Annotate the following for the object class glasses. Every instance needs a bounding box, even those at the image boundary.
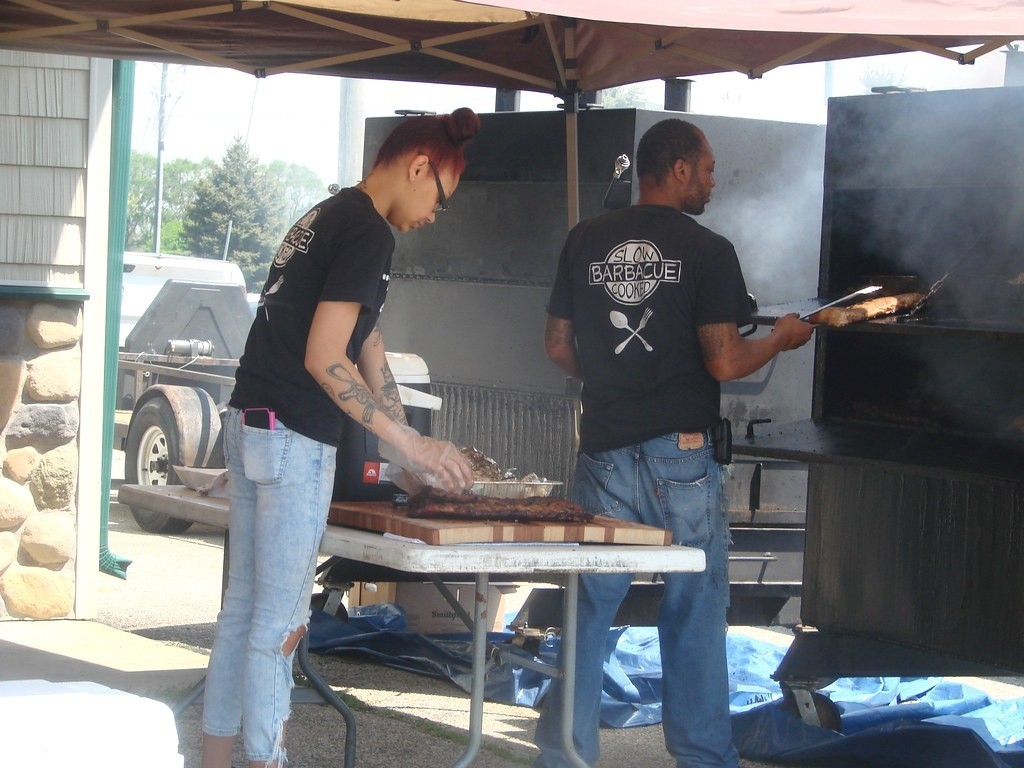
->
[418,151,450,213]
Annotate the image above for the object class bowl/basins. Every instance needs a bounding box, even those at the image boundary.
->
[171,464,227,493]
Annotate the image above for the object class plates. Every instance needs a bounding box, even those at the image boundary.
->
[471,479,563,498]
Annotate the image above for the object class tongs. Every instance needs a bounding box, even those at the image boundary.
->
[770,284,882,333]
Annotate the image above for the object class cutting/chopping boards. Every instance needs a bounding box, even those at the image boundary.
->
[324,499,673,547]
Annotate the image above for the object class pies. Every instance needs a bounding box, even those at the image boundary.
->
[808,293,924,326]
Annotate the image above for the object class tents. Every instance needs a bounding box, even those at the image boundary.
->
[0,0,1024,495]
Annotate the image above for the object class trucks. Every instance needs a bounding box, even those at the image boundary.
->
[114,253,256,533]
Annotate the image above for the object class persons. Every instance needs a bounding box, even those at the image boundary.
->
[535,120,815,768]
[199,107,480,768]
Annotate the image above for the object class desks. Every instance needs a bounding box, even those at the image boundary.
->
[116,485,708,768]
